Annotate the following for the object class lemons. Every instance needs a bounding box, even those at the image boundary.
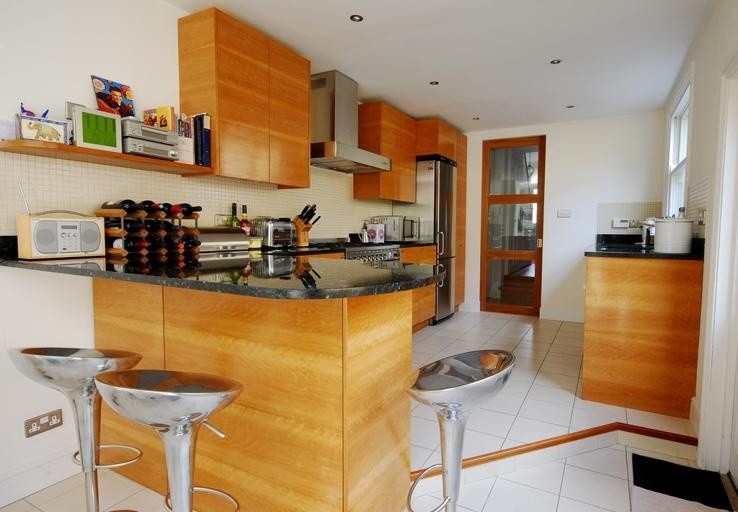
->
[357,229,372,244]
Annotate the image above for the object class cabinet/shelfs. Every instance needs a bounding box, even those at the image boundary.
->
[100,197,203,273]
[676,205,687,220]
[228,261,251,289]
[230,202,252,234]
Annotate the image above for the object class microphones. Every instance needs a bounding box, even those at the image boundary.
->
[19,346,242,510]
[405,350,517,512]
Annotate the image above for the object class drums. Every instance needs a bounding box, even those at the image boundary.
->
[295,269,322,291]
[298,202,322,228]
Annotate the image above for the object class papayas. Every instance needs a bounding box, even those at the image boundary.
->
[253,214,298,249]
[252,254,298,280]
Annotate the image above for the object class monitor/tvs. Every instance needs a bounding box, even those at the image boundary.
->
[202,115,211,168]
[194,116,203,167]
[142,106,195,138]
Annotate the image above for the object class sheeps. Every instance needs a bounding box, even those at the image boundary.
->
[634,223,651,248]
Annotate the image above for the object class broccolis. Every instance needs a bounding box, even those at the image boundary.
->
[392,153,457,327]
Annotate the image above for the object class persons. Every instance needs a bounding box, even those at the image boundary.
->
[96,86,134,117]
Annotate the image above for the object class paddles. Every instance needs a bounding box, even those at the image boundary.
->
[631,452,737,512]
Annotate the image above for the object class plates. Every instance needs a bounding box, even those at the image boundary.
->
[580,256,704,419]
[352,101,418,204]
[176,7,312,188]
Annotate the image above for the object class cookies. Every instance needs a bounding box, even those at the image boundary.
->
[370,214,420,241]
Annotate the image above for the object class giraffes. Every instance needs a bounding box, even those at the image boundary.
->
[316,241,401,260]
